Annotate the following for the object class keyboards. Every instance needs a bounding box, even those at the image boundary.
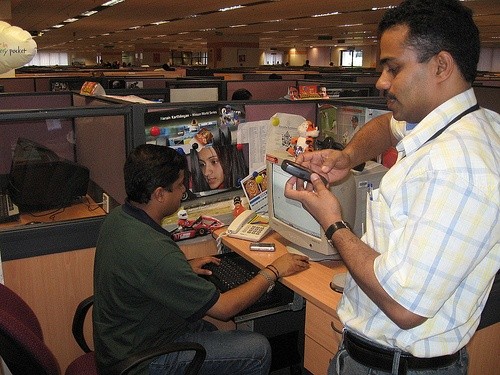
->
[0,194,20,224]
[198,253,281,313]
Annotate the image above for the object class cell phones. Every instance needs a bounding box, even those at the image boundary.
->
[281,159,327,187]
[250,242,275,252]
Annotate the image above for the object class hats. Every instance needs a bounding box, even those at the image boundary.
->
[233,196,242,204]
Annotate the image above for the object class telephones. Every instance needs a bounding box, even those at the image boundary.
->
[227,209,271,242]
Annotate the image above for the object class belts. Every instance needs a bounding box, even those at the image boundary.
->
[343,330,461,375]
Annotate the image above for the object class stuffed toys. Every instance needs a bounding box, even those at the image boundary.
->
[286,120,319,157]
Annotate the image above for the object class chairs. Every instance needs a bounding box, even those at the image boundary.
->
[0,283,206,375]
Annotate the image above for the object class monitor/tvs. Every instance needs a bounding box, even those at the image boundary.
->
[7,137,91,212]
[265,148,388,260]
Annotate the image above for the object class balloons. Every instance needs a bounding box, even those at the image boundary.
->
[0,20,37,75]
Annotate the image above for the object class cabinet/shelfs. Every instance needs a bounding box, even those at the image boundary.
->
[212,218,500,375]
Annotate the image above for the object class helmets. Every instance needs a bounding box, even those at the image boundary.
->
[178,210,188,220]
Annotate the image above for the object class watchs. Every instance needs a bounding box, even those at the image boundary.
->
[258,269,275,293]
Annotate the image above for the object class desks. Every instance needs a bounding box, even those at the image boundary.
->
[0,195,107,375]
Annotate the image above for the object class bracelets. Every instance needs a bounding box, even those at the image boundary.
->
[325,220,352,249]
[265,265,280,280]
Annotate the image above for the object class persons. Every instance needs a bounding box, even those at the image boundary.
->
[99,59,132,69]
[274,61,289,67]
[176,210,196,232]
[302,60,310,71]
[231,88,253,101]
[285,0,500,375]
[329,62,333,67]
[162,63,176,71]
[317,86,329,99]
[190,144,249,193]
[343,115,361,144]
[91,144,311,375]
[288,85,302,99]
[233,196,245,219]
